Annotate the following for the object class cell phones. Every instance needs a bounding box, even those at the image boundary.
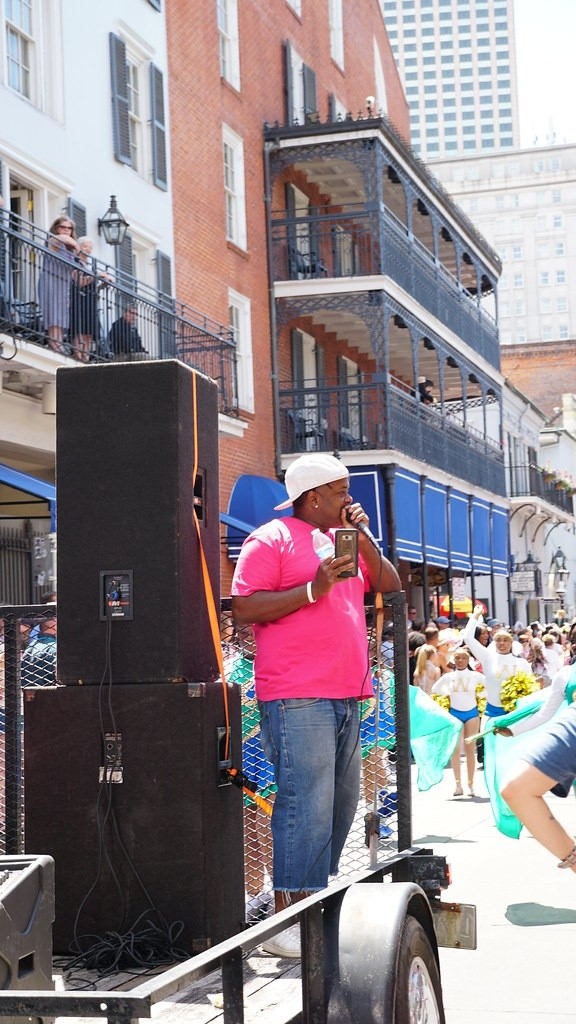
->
[335,529,358,578]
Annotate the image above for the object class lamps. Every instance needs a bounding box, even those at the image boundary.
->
[97,195,129,245]
[41,383,56,415]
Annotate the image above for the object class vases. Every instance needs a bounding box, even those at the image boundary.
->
[544,474,575,497]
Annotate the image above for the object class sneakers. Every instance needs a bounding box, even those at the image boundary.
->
[262,922,301,957]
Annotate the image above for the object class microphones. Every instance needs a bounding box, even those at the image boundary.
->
[345,508,381,553]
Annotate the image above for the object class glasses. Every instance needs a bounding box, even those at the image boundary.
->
[410,612,416,615]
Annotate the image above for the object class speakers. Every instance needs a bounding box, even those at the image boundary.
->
[57,360,222,682]
[0,854,56,1024]
[23,681,246,966]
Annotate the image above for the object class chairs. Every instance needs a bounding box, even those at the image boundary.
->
[291,247,328,279]
[286,409,376,452]
[0,281,109,362]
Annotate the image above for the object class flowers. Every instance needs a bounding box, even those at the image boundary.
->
[542,459,576,493]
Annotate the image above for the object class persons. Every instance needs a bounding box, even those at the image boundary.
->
[227,451,401,957]
[109,303,156,362]
[409,378,435,459]
[0,601,576,844]
[500,700,576,875]
[39,217,112,363]
[225,612,280,925]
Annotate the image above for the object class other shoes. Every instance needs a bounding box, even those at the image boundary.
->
[467,781,475,796]
[454,779,463,796]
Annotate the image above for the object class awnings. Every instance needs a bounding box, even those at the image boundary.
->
[0,463,57,533]
[227,472,294,564]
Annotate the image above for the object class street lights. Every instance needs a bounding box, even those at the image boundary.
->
[555,546,570,626]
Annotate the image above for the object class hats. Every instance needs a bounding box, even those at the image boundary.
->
[437,616,451,623]
[488,619,503,627]
[274,454,349,510]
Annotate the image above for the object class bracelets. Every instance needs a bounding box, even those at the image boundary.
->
[306,580,316,603]
[558,848,576,869]
